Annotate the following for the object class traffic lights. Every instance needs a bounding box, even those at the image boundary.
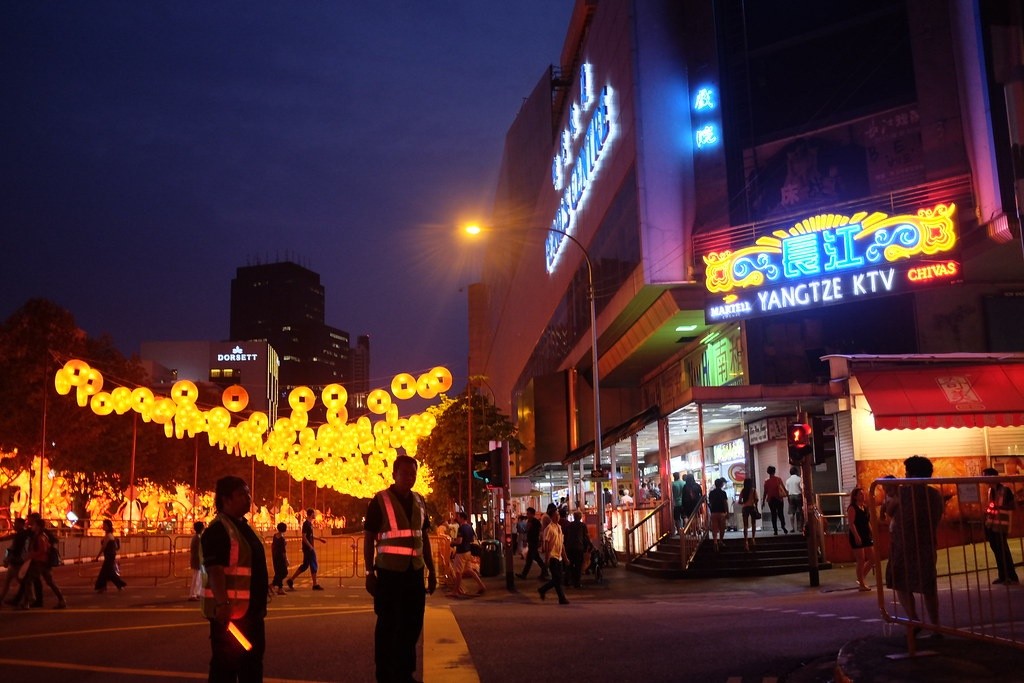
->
[810,415,836,465]
[785,421,814,467]
[471,447,503,489]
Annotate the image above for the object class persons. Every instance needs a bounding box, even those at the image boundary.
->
[448,512,487,595]
[95,519,128,593]
[603,487,612,505]
[0,512,67,609]
[477,515,505,552]
[268,522,289,595]
[671,472,702,536]
[555,497,568,508]
[785,468,804,532]
[190,521,204,601]
[617,484,633,504]
[640,481,661,501]
[882,456,944,639]
[287,509,327,590]
[512,503,593,604]
[708,477,729,552]
[761,466,788,534]
[847,488,875,591]
[738,478,759,546]
[201,476,268,683]
[364,456,437,683]
[983,468,1019,585]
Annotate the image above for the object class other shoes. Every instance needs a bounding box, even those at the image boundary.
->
[538,586,546,601]
[855,578,871,592]
[188,595,200,602]
[559,600,570,606]
[914,626,946,639]
[268,579,295,596]
[97,586,108,594]
[744,538,757,552]
[54,600,65,610]
[312,584,325,590]
[774,532,778,535]
[713,540,726,554]
[790,529,795,533]
[781,526,788,534]
[515,572,526,578]
[118,583,128,593]
[475,587,488,595]
[537,572,550,580]
[574,584,581,588]
[445,592,461,598]
[0,597,45,609]
[992,577,1020,585]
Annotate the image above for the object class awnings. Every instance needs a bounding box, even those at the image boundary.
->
[854,363,1024,431]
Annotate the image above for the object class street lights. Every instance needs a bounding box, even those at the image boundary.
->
[461,217,607,566]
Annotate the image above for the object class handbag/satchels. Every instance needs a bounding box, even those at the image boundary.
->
[115,538,120,550]
[470,543,482,556]
[752,500,762,519]
[777,482,787,498]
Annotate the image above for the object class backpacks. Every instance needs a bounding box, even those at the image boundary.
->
[43,533,63,568]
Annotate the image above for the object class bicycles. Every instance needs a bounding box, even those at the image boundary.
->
[599,525,619,569]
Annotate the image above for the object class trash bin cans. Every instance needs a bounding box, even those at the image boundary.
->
[480,541,501,577]
